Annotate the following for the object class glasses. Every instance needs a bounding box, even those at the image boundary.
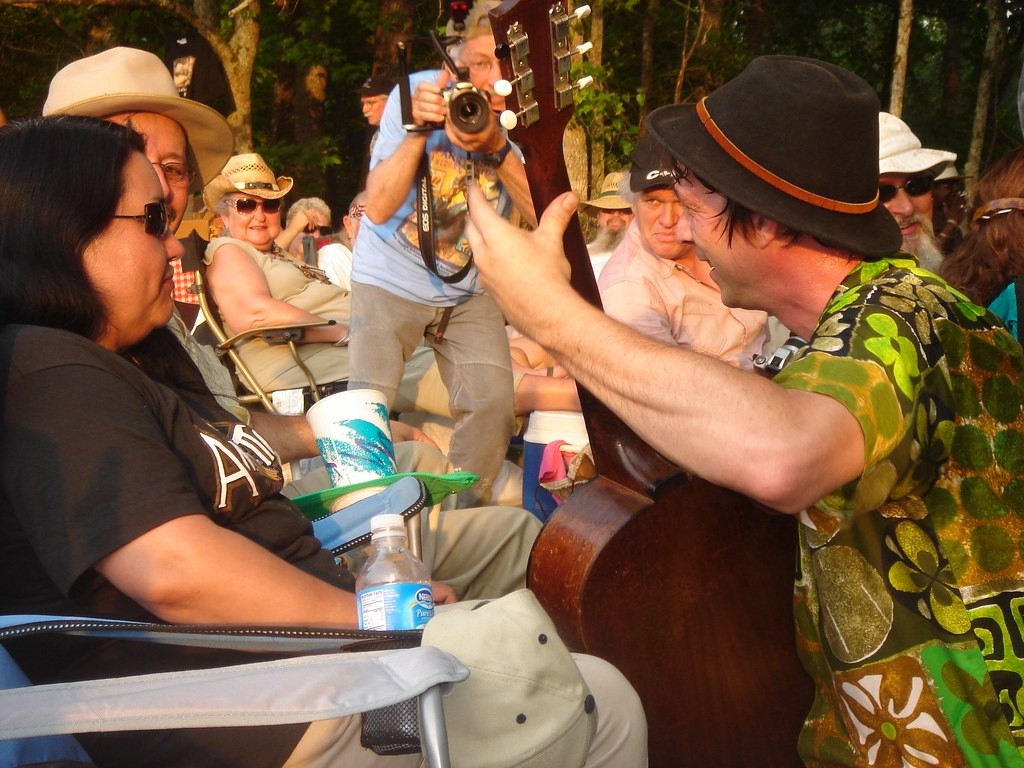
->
[150,161,196,190]
[878,174,938,202]
[113,201,169,238]
[223,198,281,215]
[601,208,633,217]
[302,223,333,237]
[935,179,957,186]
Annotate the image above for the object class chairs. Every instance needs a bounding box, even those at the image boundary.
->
[196,268,338,416]
[0,456,482,767]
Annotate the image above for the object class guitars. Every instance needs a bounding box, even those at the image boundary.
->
[483,0,815,768]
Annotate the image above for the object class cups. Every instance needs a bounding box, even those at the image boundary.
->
[306,389,398,489]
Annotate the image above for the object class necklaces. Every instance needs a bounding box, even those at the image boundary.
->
[262,241,331,285]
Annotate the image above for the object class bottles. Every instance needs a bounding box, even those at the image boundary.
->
[357,514,435,753]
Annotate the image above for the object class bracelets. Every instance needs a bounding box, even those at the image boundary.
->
[334,329,350,346]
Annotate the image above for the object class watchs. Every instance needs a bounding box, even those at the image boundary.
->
[485,140,512,167]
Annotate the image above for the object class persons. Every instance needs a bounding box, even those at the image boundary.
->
[200,153,634,502]
[466,58,1024,768]
[0,116,648,768]
[879,112,1023,348]
[347,0,538,509]
[44,47,543,601]
[599,137,806,370]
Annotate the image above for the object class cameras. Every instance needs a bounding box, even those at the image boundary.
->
[427,66,492,134]
[752,330,810,381]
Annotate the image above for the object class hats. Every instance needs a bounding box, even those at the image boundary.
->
[203,153,294,214]
[578,172,632,218]
[422,587,597,768]
[924,160,974,181]
[353,76,393,96]
[444,0,505,56]
[43,47,235,195]
[877,110,958,179]
[630,139,675,195]
[646,54,903,260]
